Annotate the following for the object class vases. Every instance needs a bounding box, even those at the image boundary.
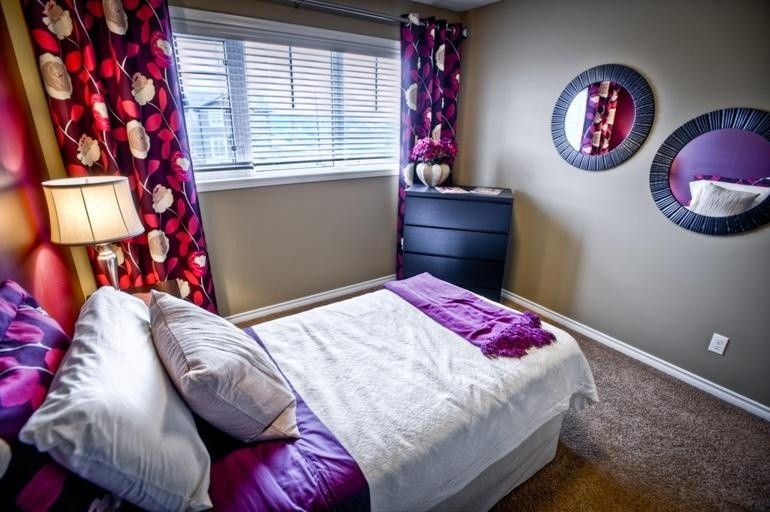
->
[415,163,451,192]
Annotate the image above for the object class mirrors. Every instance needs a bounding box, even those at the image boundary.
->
[549,62,770,236]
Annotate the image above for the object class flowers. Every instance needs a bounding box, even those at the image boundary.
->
[408,136,460,163]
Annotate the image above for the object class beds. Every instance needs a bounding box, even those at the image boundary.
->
[120,272,585,510]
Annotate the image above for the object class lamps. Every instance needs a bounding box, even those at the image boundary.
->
[41,176,147,291]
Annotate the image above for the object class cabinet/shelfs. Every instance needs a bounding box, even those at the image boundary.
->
[401,185,514,302]
